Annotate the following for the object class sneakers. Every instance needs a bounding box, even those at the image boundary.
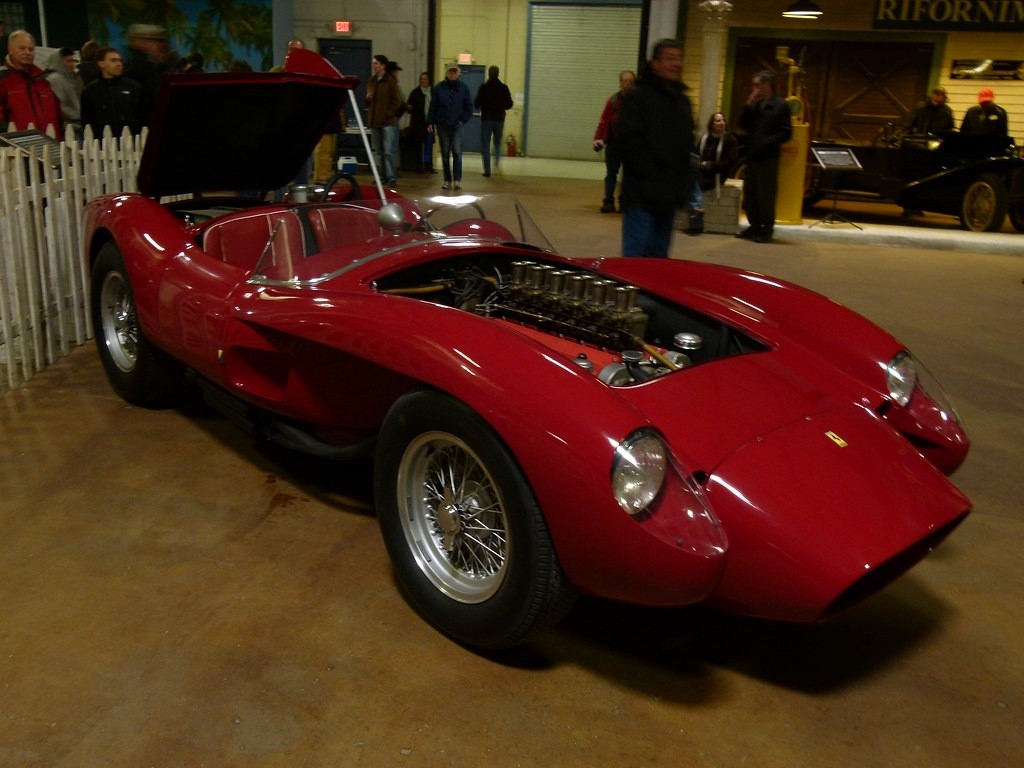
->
[442,181,451,189]
[455,180,461,189]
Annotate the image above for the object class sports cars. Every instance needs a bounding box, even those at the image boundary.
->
[85,48,975,654]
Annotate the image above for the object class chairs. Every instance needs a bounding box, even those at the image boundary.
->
[201,207,383,269]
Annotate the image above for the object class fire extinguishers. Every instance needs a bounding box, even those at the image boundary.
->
[506,134,518,157]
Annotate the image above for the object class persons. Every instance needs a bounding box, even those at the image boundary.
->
[613,37,696,259]
[0,20,9,66]
[736,71,793,244]
[901,85,954,218]
[593,70,637,213]
[75,41,102,86]
[231,59,253,72]
[427,62,473,189]
[364,54,406,188]
[960,88,1008,136]
[408,72,438,174]
[80,47,138,150]
[474,65,513,177]
[174,52,209,73]
[0,30,63,143]
[44,48,86,140]
[687,112,738,235]
[128,22,172,90]
[269,36,305,72]
[313,107,346,186]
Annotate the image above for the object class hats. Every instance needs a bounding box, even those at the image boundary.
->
[979,88,993,103]
[447,63,458,71]
[386,61,402,71]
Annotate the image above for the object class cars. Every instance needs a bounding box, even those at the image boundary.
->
[805,123,1024,237]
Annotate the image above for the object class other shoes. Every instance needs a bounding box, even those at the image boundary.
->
[390,181,396,188]
[417,168,438,174]
[380,178,389,183]
[601,201,615,212]
[736,225,772,242]
[692,208,705,216]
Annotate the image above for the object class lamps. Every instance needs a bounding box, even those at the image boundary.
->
[698,0,733,23]
